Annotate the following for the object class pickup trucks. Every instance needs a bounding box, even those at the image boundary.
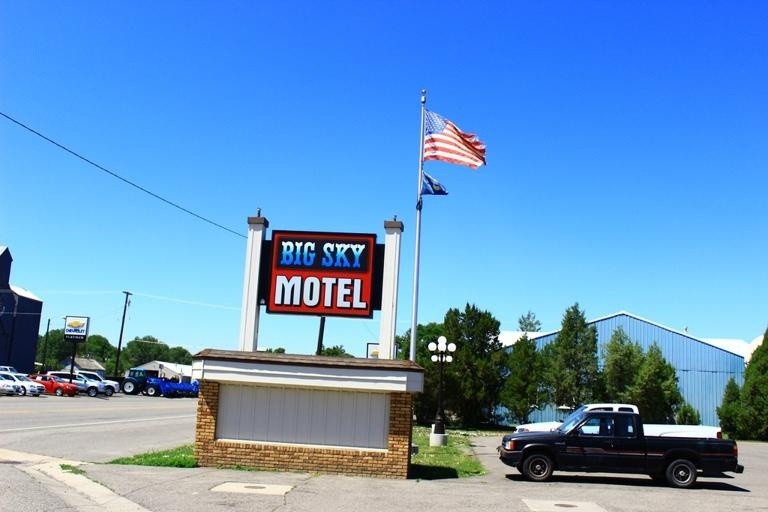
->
[495,400,746,489]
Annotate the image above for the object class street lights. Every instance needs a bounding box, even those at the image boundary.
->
[112,287,133,378]
[428,335,457,447]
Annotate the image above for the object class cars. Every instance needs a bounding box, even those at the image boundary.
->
[0,363,121,404]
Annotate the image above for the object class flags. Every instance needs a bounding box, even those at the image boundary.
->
[421,171,448,195]
[422,108,487,170]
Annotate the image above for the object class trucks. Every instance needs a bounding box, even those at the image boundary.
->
[122,368,199,398]
